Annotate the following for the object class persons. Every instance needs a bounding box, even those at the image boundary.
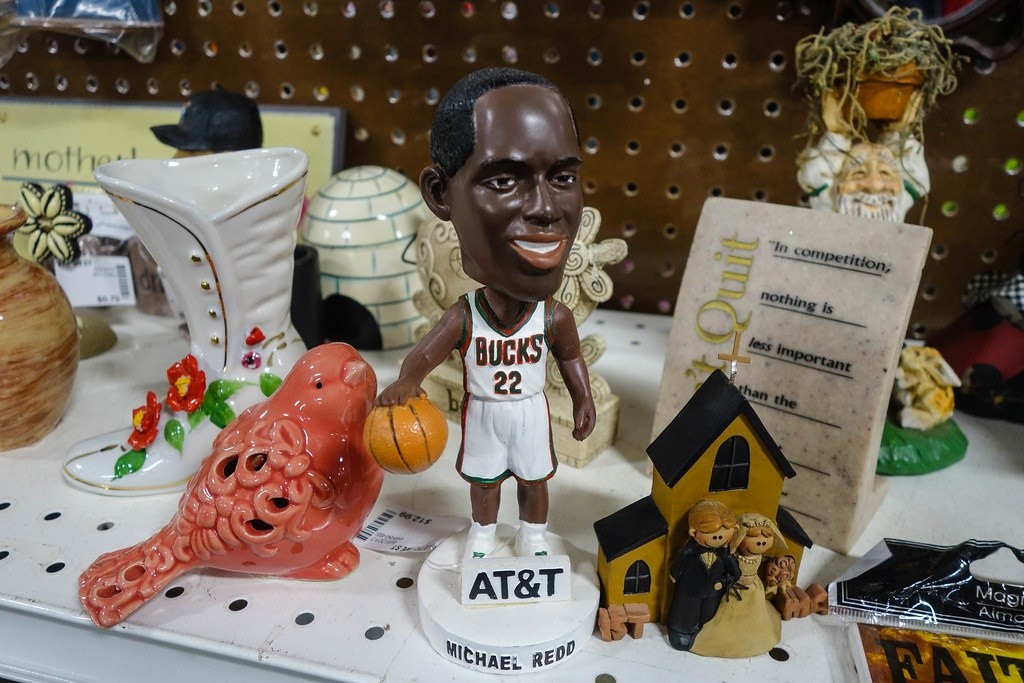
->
[797,133,931,222]
[664,499,743,647]
[688,514,787,658]
[148,93,313,242]
[376,67,595,564]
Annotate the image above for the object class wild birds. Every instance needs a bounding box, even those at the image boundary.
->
[79,342,386,627]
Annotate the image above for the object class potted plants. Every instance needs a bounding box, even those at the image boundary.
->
[794,7,960,121]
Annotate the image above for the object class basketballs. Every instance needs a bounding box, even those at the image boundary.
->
[362,398,450,475]
